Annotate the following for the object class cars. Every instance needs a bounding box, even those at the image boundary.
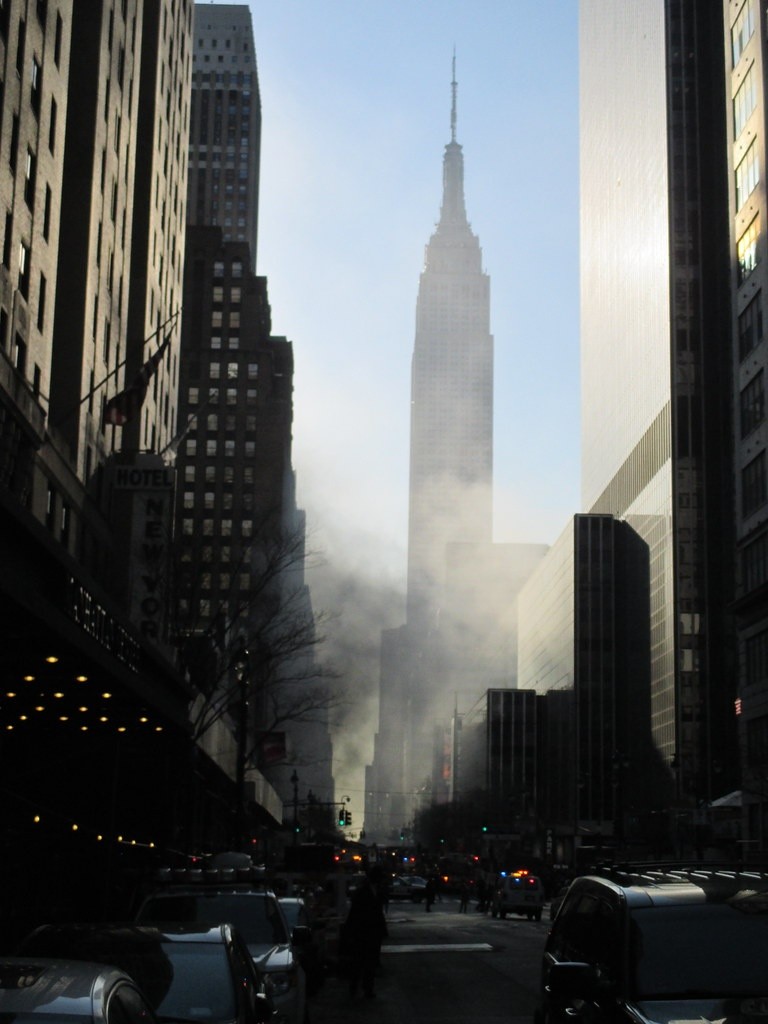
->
[276,895,320,995]
[133,884,311,1024]
[368,848,474,893]
[348,873,368,896]
[0,956,161,1024]
[334,847,361,867]
[383,875,430,903]
[10,919,290,1024]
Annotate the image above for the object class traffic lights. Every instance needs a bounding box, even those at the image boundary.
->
[345,811,351,826]
[339,810,344,825]
[400,832,404,841]
[481,825,488,832]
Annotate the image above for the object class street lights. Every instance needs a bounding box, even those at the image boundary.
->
[611,749,631,852]
[670,753,697,846]
[575,777,584,836]
[291,769,300,818]
[521,790,527,814]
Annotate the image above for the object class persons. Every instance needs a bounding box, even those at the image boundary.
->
[459,883,469,913]
[335,885,389,997]
[425,879,433,912]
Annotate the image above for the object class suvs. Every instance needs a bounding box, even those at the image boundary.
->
[491,869,545,921]
[534,864,768,1024]
